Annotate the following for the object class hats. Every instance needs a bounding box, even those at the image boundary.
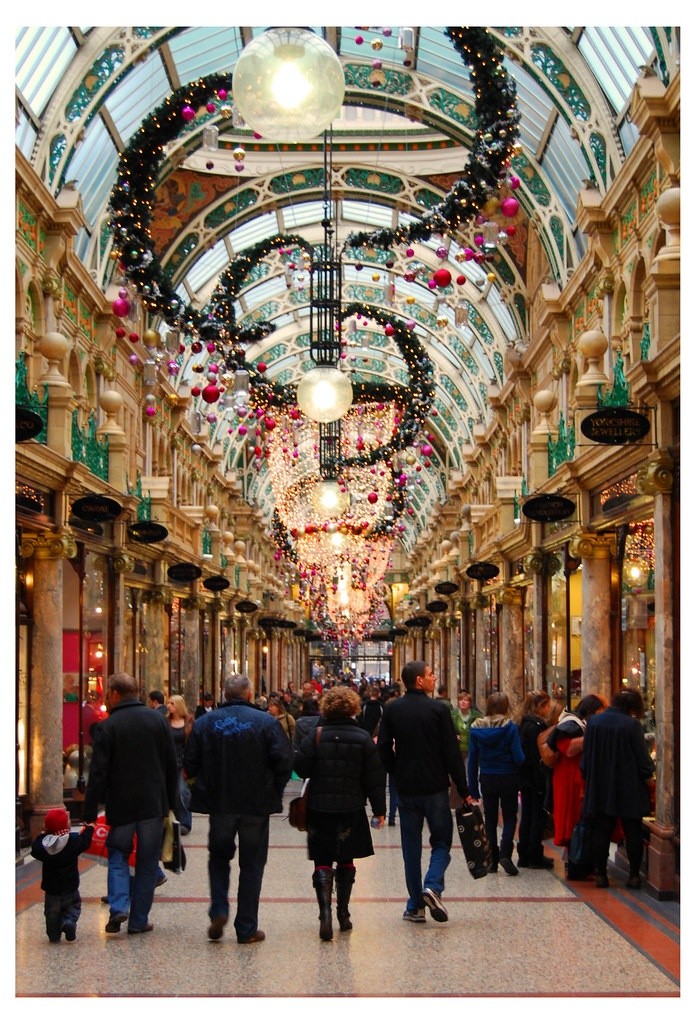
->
[41,808,69,835]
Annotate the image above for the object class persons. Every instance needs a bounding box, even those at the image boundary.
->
[467,692,525,875]
[166,695,193,835]
[388,782,398,826]
[183,674,294,943]
[30,809,95,944]
[196,694,212,717]
[257,672,401,743]
[83,673,180,934]
[580,687,655,890]
[436,685,453,710]
[514,690,554,869]
[148,690,168,716]
[554,694,604,847]
[450,692,485,810]
[293,686,386,940]
[377,661,472,923]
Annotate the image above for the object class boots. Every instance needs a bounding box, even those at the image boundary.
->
[312,866,334,941]
[334,864,355,931]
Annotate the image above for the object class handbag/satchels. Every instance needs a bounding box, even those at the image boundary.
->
[455,801,492,880]
[159,812,186,875]
[566,799,586,861]
[537,723,563,769]
[287,797,306,831]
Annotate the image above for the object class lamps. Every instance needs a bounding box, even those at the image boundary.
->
[296,123,353,553]
[231,27,346,144]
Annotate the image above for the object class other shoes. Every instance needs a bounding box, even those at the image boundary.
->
[492,840,645,891]
[48,934,62,943]
[155,872,168,888]
[61,920,77,941]
[237,931,265,944]
[127,920,154,935]
[207,915,228,939]
[387,816,396,827]
[402,907,426,922]
[105,912,129,933]
[422,887,448,922]
[101,895,109,904]
